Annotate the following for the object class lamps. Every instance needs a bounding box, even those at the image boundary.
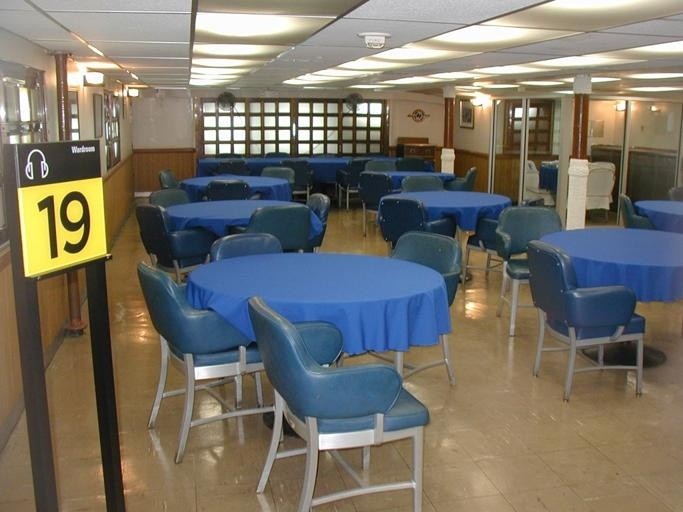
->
[468,97,483,106]
[127,86,139,98]
[79,69,106,87]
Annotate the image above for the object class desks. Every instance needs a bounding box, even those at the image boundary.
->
[179,251,450,437]
[539,158,557,200]
[631,199,683,235]
[380,191,511,230]
[534,228,683,368]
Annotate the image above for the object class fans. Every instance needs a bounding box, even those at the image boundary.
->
[344,92,366,113]
[214,90,235,112]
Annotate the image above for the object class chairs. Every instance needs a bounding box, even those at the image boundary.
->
[246,291,431,511]
[468,219,504,280]
[230,206,311,252]
[135,204,215,282]
[132,260,292,463]
[522,158,551,209]
[584,161,615,224]
[520,239,648,404]
[206,233,282,264]
[662,187,681,205]
[378,196,456,250]
[383,229,469,389]
[495,207,561,336]
[149,152,477,247]
[617,188,644,229]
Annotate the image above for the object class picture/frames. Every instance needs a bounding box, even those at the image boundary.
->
[458,98,476,132]
